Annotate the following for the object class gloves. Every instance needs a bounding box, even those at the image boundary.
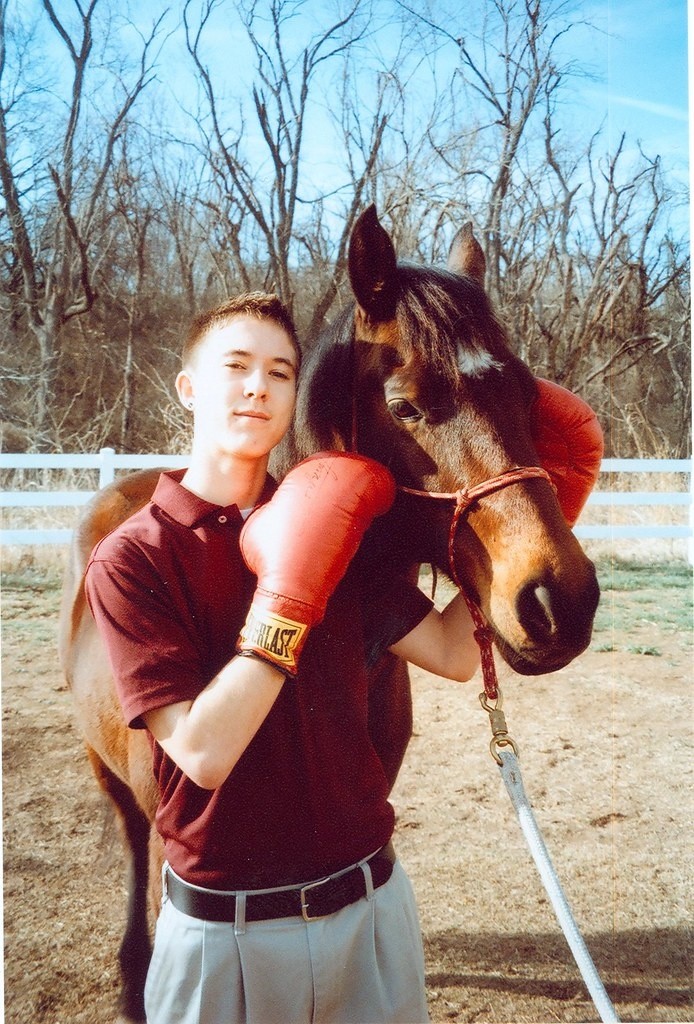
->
[527,377,604,528]
[240,448,395,677]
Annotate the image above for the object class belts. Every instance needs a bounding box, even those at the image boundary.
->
[167,841,397,923]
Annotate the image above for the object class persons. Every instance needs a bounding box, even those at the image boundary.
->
[84,293,604,1024]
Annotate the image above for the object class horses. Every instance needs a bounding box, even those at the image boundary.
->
[64,203,597,1024]
